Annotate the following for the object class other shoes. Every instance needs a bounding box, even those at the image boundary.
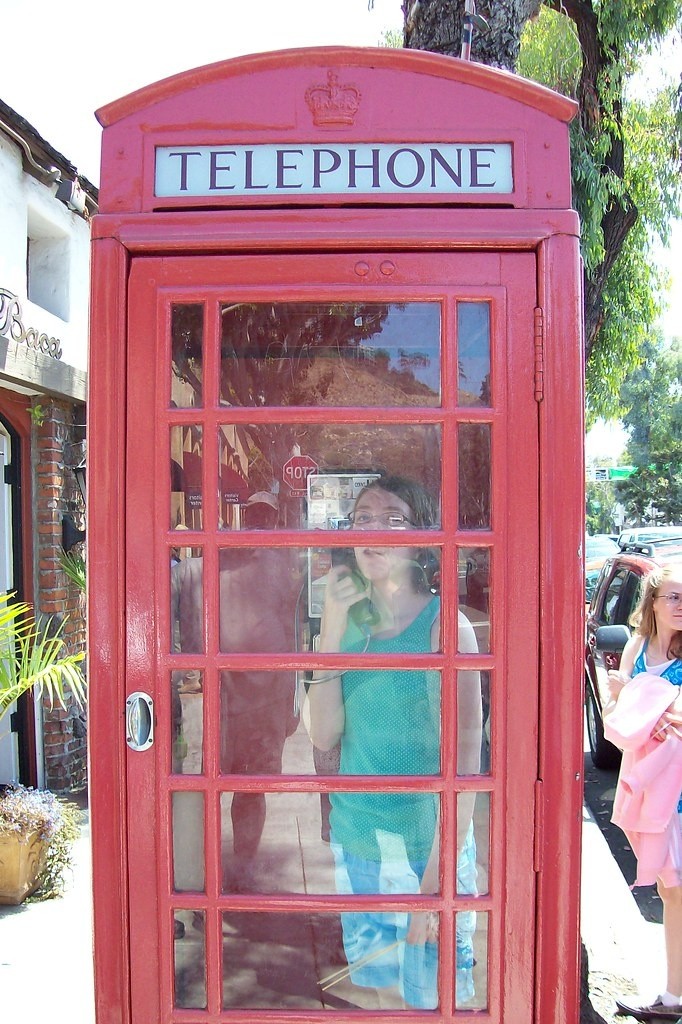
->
[177,681,201,693]
[615,994,682,1020]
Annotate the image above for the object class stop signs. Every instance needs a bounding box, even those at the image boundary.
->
[282,455,319,490]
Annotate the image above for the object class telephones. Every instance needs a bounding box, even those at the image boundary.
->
[330,544,381,630]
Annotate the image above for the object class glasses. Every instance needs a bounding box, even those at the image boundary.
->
[654,594,682,604]
[347,510,420,529]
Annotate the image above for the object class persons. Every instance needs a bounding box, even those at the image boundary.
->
[600,563,682,1021]
[168,490,299,940]
[303,475,486,1014]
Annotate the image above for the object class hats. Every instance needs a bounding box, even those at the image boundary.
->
[244,490,280,513]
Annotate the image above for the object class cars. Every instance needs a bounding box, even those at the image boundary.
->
[582,521,682,770]
[415,549,440,593]
[463,546,494,611]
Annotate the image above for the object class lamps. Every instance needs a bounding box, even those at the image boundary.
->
[62,452,86,555]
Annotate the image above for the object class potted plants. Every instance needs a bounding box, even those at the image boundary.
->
[0,779,86,905]
